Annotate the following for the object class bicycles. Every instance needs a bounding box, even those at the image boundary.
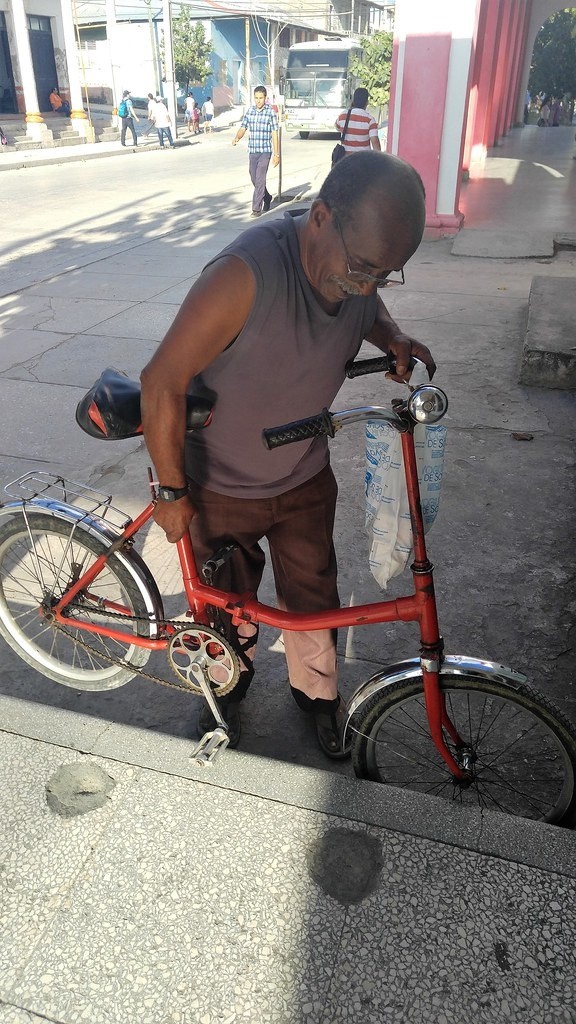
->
[0,354,576,829]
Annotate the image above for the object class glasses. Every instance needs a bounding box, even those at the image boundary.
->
[326,201,405,288]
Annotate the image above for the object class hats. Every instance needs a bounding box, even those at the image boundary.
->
[124,90,131,94]
[156,96,164,101]
[355,88,369,105]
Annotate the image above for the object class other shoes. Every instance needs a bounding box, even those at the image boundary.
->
[263,195,272,211]
[314,690,359,758]
[197,697,241,749]
[250,210,261,217]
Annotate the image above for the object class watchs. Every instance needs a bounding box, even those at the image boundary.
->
[274,154,280,158]
[158,486,189,502]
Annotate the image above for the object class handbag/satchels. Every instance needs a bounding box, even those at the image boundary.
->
[332,144,345,166]
[364,362,447,590]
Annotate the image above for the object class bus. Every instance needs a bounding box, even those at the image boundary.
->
[279,36,388,139]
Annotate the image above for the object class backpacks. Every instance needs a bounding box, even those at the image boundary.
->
[118,99,128,117]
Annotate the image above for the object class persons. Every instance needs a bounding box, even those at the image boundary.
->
[141,92,156,138]
[191,102,201,135]
[232,86,279,218]
[0,127,8,145]
[334,88,381,158]
[137,152,438,756]
[539,97,561,128]
[201,96,215,134]
[525,85,532,125]
[151,96,176,147]
[49,87,70,117]
[185,92,195,132]
[121,90,140,147]
